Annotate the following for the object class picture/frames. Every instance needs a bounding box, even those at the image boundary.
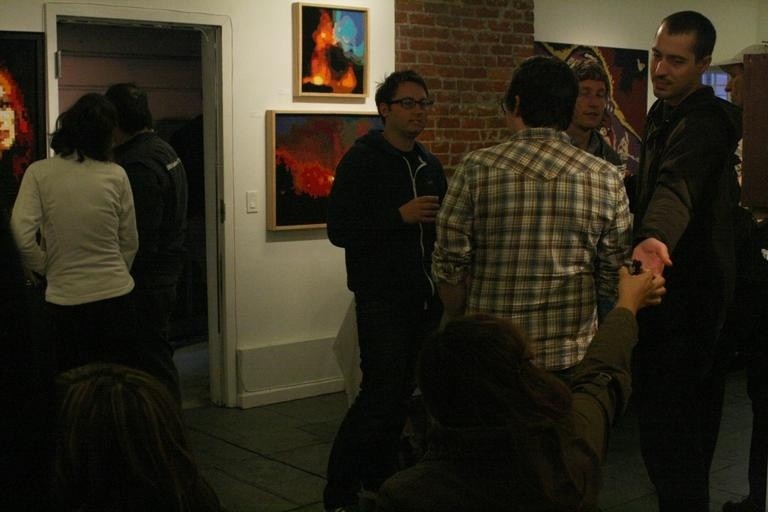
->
[265,109,383,231]
[290,2,369,100]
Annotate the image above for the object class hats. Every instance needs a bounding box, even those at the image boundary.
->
[717,43,768,76]
[554,45,614,102]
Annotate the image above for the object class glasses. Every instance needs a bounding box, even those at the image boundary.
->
[391,95,434,111]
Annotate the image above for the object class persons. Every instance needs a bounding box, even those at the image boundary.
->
[29,363,219,510]
[565,59,621,167]
[325,70,448,511]
[9,93,147,371]
[374,265,666,510]
[104,83,191,408]
[431,54,635,382]
[1,68,32,187]
[630,11,741,508]
[723,220,767,512]
